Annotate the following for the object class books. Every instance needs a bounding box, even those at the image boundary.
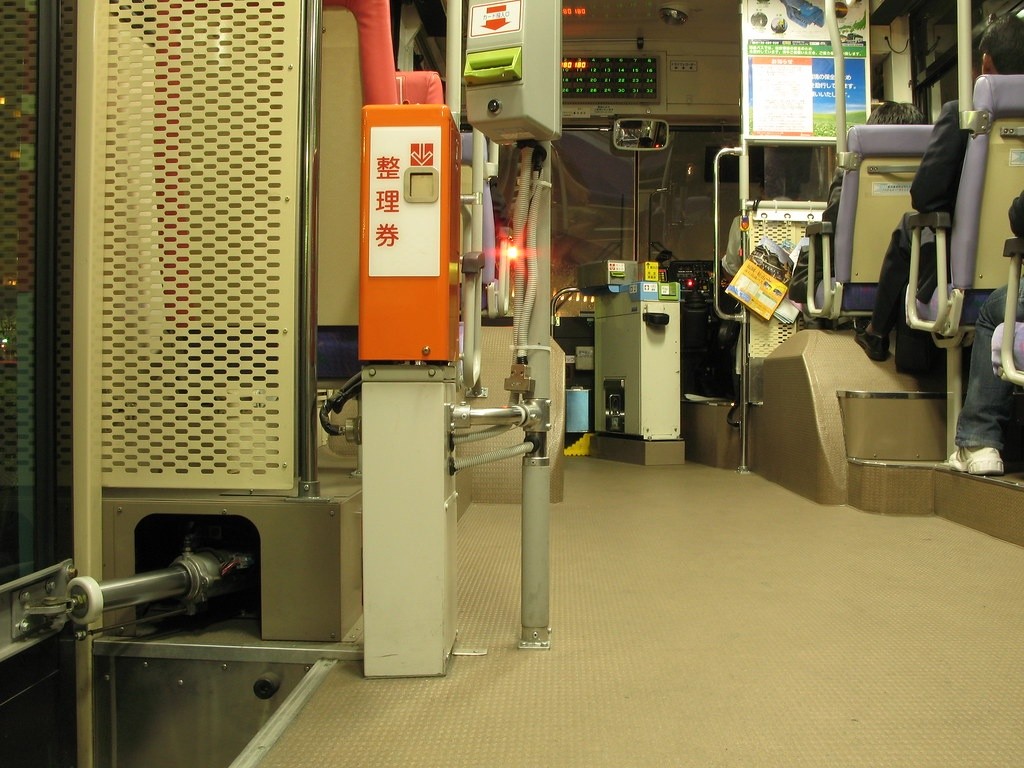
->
[725,237,794,321]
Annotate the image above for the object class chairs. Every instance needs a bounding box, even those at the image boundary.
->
[805,124,938,321]
[904,74,1024,464]
[990,237,1024,386]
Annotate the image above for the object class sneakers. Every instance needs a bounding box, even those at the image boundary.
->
[948,446,1004,476]
[854,319,891,361]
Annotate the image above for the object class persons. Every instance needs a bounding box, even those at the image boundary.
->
[721,154,804,285]
[858,14,1024,363]
[791,104,926,329]
[950,192,1024,475]
